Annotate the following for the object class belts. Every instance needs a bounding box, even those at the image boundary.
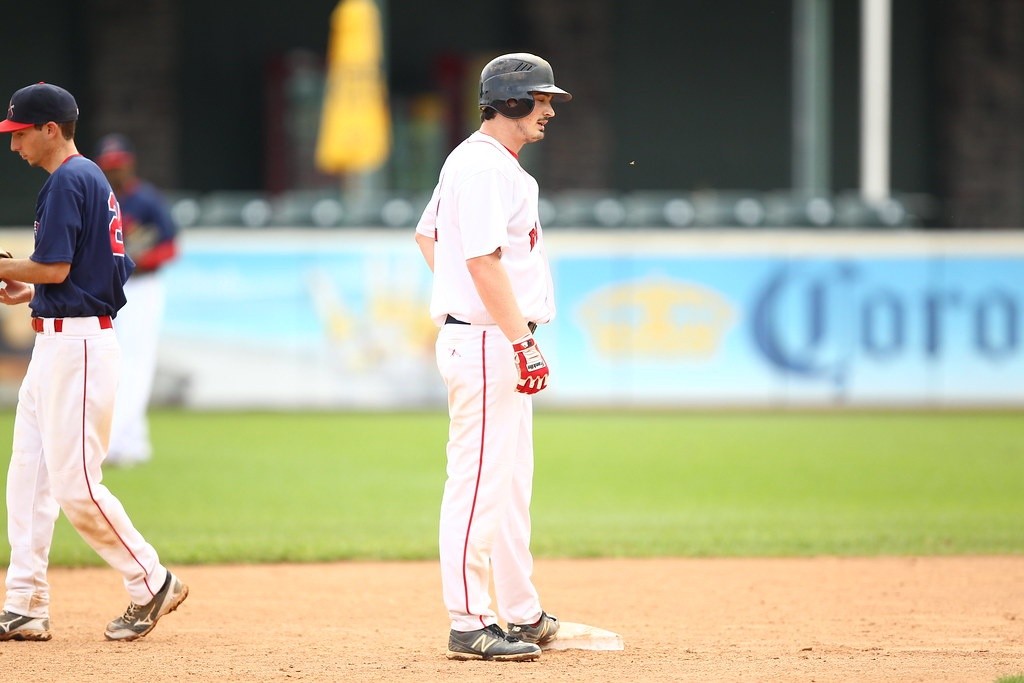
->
[30,315,113,334]
[445,315,536,335]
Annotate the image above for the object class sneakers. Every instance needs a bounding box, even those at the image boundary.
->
[505,610,560,644]
[104,568,187,641]
[446,624,542,663]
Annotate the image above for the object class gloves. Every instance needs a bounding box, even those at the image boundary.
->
[511,333,550,394]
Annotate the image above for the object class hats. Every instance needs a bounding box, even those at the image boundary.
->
[0,82,79,133]
[96,133,140,169]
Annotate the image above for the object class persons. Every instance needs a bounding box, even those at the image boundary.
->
[97,132,180,466]
[414,53,572,661]
[0,81,190,642]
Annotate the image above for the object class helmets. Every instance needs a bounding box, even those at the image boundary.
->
[479,52,573,119]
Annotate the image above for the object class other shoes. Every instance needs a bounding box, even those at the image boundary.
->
[0,610,53,641]
